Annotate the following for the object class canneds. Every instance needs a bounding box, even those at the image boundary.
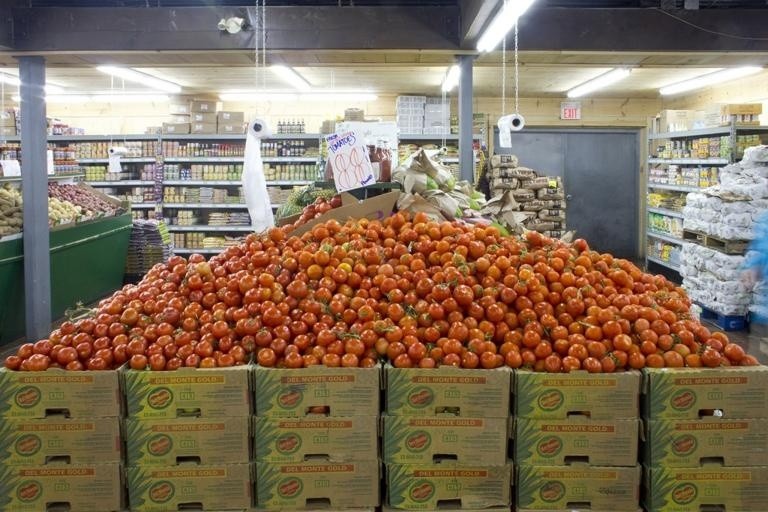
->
[83,164,243,180]
[68,140,157,159]
[163,186,185,203]
[118,187,143,204]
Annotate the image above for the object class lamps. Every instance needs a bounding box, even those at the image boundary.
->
[565,66,630,98]
[659,66,762,96]
[218,16,245,34]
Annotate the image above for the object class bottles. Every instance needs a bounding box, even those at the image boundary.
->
[47,117,81,175]
[177,115,308,157]
[0,142,22,167]
[366,138,395,184]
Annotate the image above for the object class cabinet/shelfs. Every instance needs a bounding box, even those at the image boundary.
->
[643,125,768,285]
[0,213,136,345]
[0,134,483,254]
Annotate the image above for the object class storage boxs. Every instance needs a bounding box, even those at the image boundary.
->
[254,418,380,462]
[125,418,252,465]
[516,464,645,510]
[3,420,125,469]
[3,466,129,509]
[256,367,383,418]
[128,468,257,510]
[645,417,766,469]
[641,366,768,420]
[380,415,516,467]
[383,463,517,511]
[510,365,646,419]
[162,101,246,133]
[396,95,451,134]
[2,365,127,420]
[257,462,382,512]
[122,365,254,420]
[385,368,513,420]
[643,463,766,510]
[512,417,649,472]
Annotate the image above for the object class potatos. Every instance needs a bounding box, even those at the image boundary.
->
[0,190,23,237]
[49,181,116,213]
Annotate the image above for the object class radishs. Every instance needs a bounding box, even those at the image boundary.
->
[0,187,93,228]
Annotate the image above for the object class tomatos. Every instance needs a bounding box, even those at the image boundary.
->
[435,407,459,413]
[151,391,169,405]
[675,438,695,452]
[279,437,297,450]
[413,484,432,498]
[152,484,171,500]
[310,405,326,414]
[152,438,170,452]
[674,392,693,406]
[19,438,37,451]
[19,390,37,404]
[541,393,560,407]
[20,484,38,498]
[675,487,694,500]
[543,485,561,498]
[540,439,560,453]
[280,393,300,405]
[281,480,299,495]
[411,391,430,404]
[6,196,759,372]
[409,434,427,448]
